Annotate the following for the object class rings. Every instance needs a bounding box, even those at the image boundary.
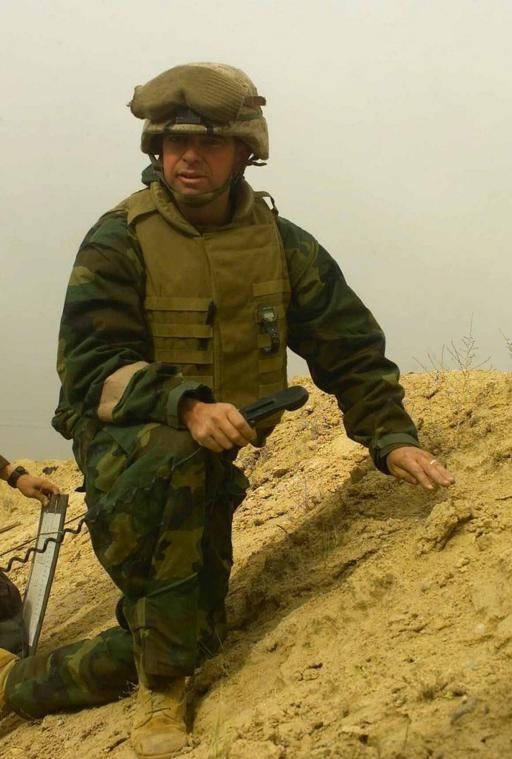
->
[429,459,437,465]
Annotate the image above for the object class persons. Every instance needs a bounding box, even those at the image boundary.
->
[0,446,71,659]
[0,62,456,759]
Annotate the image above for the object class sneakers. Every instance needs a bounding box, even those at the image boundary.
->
[131,674,189,759]
[0,648,22,724]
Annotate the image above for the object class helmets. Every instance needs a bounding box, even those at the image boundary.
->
[141,61,270,160]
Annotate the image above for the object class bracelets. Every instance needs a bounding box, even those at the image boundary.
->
[7,466,29,489]
